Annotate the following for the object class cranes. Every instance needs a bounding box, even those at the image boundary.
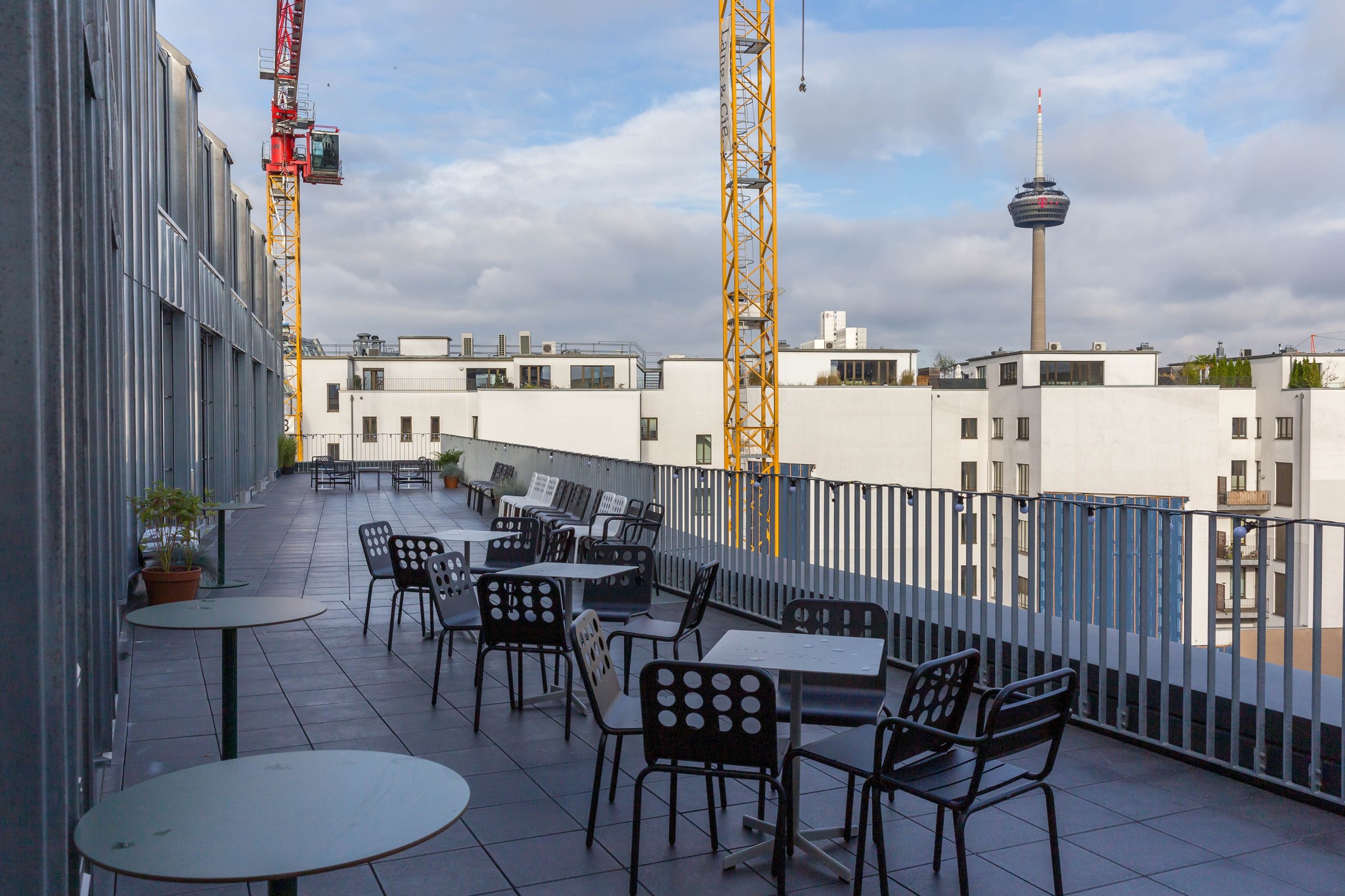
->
[717,0,780,562]
[253,0,348,463]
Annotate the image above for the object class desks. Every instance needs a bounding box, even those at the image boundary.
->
[419,530,523,643]
[495,562,639,717]
[197,503,267,589]
[397,468,421,489]
[72,748,471,895]
[358,467,380,490]
[125,596,327,761]
[310,469,334,490]
[700,629,884,883]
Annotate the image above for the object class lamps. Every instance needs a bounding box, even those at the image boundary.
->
[459,367,463,371]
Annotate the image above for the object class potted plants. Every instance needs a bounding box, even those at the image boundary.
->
[430,447,465,488]
[489,473,528,517]
[748,367,774,386]
[278,432,300,478]
[814,369,842,386]
[127,479,224,606]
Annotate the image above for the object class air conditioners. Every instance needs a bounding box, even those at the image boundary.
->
[1092,342,1106,351]
[825,341,837,349]
[542,342,556,355]
[1048,342,1061,350]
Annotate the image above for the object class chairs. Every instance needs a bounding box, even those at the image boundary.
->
[358,456,1077,896]
[311,456,356,493]
[1250,549,1258,559]
